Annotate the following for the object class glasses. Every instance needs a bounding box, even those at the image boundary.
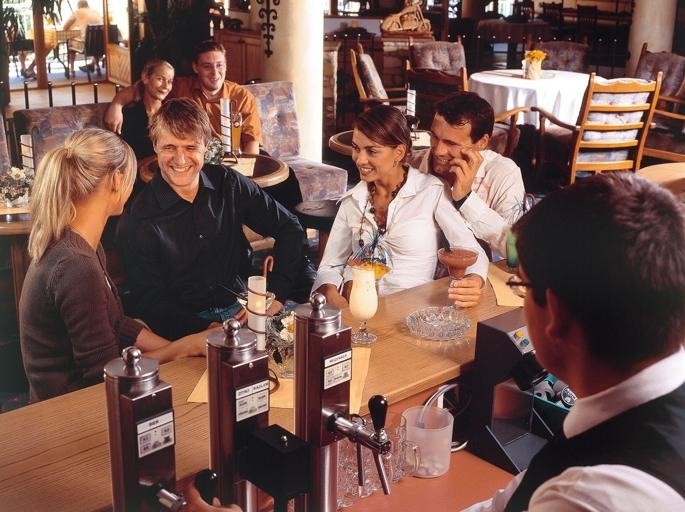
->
[504,274,535,300]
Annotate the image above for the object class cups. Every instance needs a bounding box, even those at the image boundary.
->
[230,111,242,157]
[336,406,455,507]
[505,226,520,275]
[235,290,277,314]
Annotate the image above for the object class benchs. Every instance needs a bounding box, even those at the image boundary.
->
[12,81,347,203]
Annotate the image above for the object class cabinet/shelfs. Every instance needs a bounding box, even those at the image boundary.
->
[213,29,262,85]
[107,44,131,88]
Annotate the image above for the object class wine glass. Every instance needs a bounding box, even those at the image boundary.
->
[435,245,480,309]
[346,267,381,343]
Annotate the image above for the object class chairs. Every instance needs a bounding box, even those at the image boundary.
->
[634,42,685,162]
[531,71,663,185]
[12,239,117,405]
[5,24,120,81]
[349,36,529,158]
[476,0,620,69]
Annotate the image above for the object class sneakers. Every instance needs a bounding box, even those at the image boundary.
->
[95,66,102,77]
[79,63,95,74]
[20,68,37,80]
[69,69,76,78]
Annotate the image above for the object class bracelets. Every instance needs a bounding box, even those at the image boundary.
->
[454,190,471,209]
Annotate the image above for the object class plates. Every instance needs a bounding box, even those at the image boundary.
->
[406,306,474,340]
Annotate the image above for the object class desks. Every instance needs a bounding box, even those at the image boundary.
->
[0,200,35,236]
[138,154,289,190]
[328,127,431,156]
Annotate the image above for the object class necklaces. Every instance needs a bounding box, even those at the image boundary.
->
[357,166,409,256]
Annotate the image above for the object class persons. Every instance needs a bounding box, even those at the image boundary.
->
[309,105,489,309]
[17,127,223,405]
[106,97,315,340]
[116,60,175,197]
[19,0,129,78]
[413,90,525,279]
[104,39,302,210]
[465,171,685,512]
